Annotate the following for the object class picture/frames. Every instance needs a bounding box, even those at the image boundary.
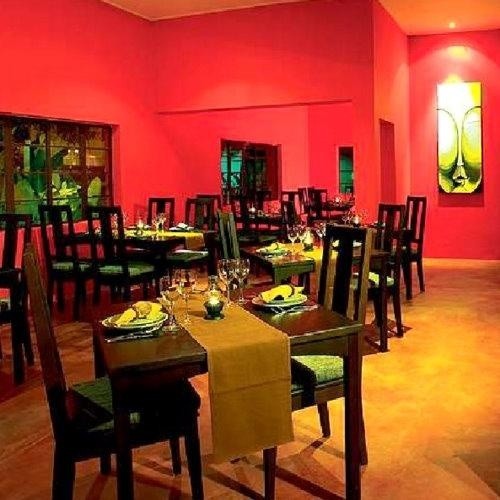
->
[436,80,484,194]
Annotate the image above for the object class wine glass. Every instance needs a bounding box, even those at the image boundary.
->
[235,260,252,301]
[108,206,172,234]
[176,269,195,324]
[286,222,332,248]
[218,258,238,306]
[159,276,182,332]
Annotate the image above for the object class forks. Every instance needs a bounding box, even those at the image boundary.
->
[105,325,168,342]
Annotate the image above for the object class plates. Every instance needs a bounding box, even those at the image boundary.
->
[102,314,168,329]
[253,296,310,307]
[333,242,363,249]
